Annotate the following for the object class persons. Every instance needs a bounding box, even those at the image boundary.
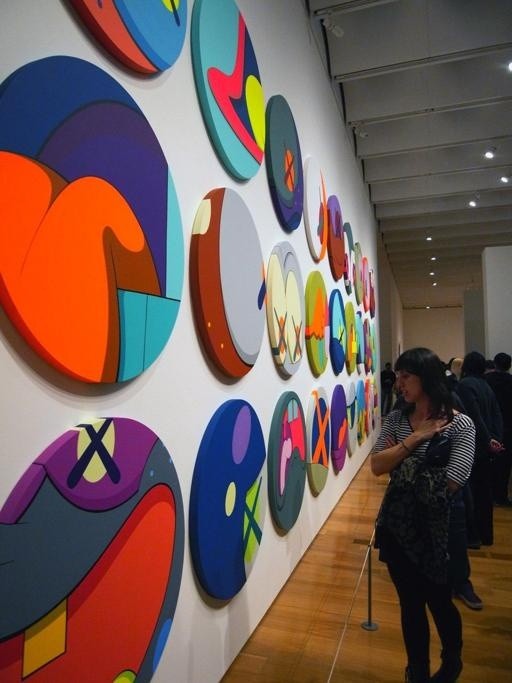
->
[380,352,511,610]
[368,347,477,682]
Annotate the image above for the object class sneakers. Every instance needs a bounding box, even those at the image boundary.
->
[454,580,483,608]
[406,657,463,682]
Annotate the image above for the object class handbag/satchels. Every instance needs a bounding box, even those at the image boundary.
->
[425,431,450,465]
